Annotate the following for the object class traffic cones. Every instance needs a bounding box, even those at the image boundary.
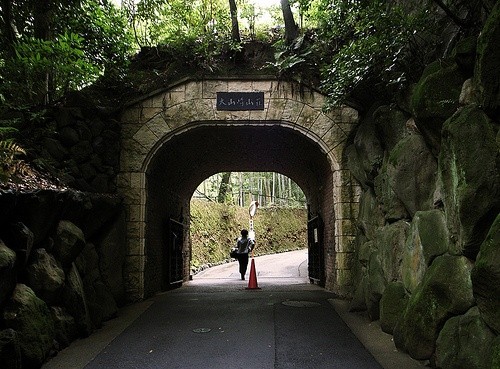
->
[245,259,261,289]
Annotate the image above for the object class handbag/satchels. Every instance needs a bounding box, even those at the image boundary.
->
[230,247,238,258]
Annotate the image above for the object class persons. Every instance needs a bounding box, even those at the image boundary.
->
[237,230,255,281]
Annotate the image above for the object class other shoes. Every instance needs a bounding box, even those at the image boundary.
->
[241,272,245,280]
